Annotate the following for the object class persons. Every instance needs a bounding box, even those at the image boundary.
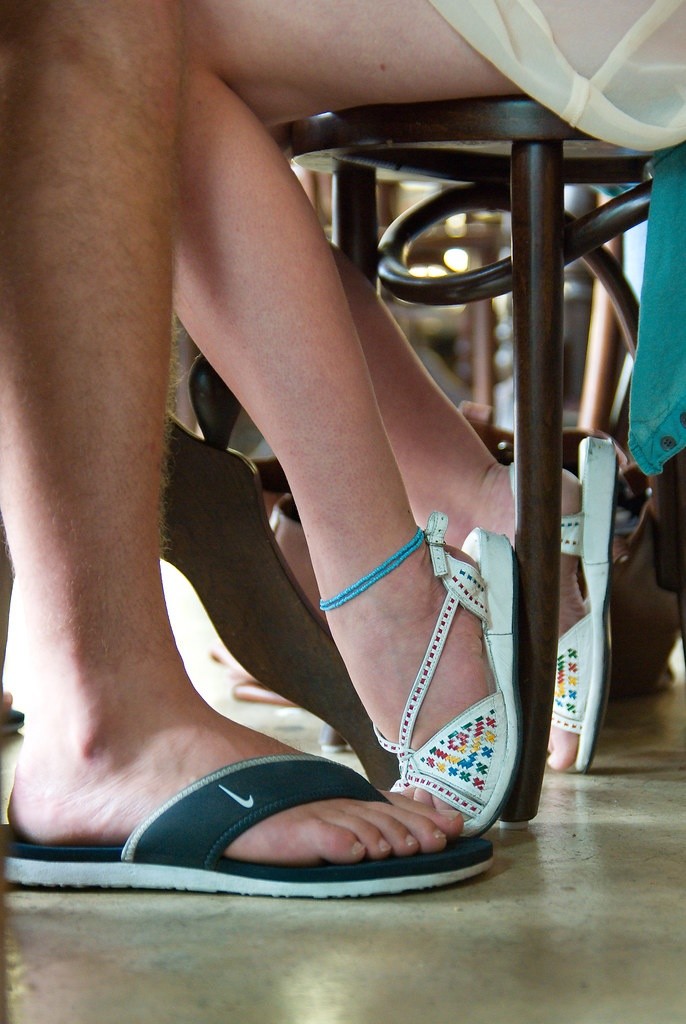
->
[0,0,686,902]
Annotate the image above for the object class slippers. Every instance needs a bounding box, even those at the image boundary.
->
[4,751,493,897]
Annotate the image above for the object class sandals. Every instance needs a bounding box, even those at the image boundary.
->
[317,512,519,837]
[510,433,617,773]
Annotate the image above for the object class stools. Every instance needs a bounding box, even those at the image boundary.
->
[189,93,686,824]
